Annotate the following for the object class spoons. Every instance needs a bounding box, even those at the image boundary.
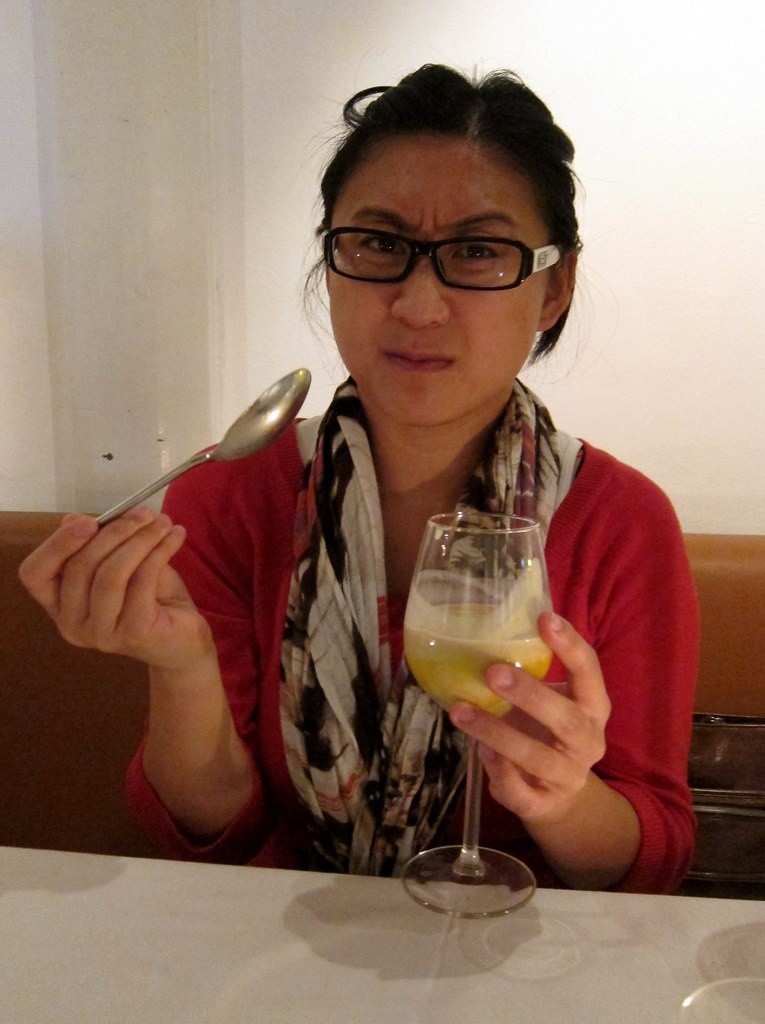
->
[90,366,312,528]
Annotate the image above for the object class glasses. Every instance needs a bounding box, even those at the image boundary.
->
[324,224,564,290]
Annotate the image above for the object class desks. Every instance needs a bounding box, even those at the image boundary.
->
[0,846,765,1024]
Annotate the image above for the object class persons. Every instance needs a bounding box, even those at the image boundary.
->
[16,64,699,893]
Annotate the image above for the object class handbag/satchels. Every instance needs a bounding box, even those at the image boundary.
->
[682,712,765,886]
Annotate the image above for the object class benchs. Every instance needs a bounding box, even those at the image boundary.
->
[0,508,765,899]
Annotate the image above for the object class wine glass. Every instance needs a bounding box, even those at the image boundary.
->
[403,510,555,921]
